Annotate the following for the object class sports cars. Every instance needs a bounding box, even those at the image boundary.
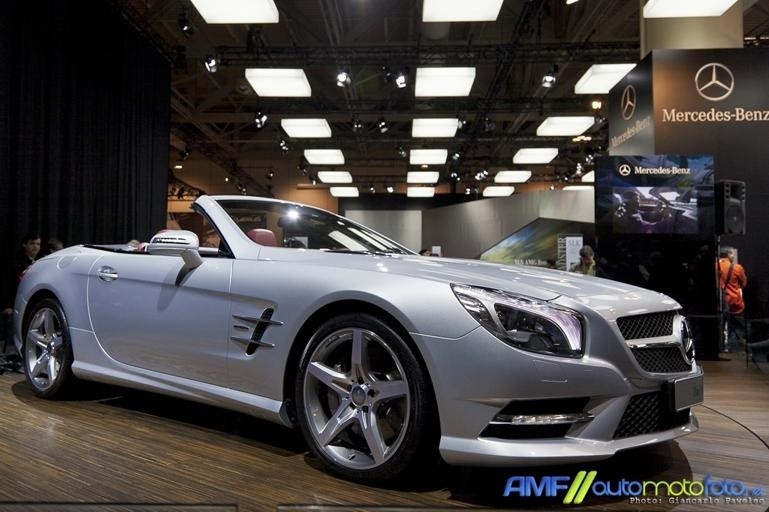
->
[14,196,704,488]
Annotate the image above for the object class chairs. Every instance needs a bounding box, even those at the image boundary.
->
[248,228,277,247]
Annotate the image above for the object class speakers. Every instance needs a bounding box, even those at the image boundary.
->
[715,179,747,236]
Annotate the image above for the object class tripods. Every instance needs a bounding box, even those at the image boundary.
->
[717,234,760,370]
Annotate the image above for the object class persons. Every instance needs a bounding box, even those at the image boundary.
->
[0,234,63,315]
[569,246,597,276]
[719,247,747,340]
[419,249,431,256]
[547,259,557,269]
[622,190,672,234]
